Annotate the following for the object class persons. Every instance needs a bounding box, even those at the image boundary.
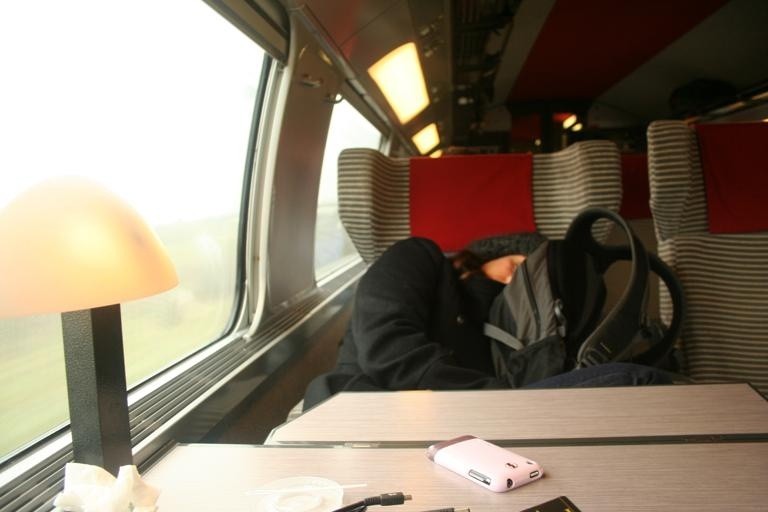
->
[304,230,549,411]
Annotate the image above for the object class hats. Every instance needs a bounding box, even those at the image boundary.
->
[463,232,547,271]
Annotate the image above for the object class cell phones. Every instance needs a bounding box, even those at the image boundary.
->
[426,434,544,493]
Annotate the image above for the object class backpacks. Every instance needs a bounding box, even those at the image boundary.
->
[482,207,681,387]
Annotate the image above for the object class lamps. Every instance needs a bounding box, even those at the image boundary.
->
[0,176,181,478]
